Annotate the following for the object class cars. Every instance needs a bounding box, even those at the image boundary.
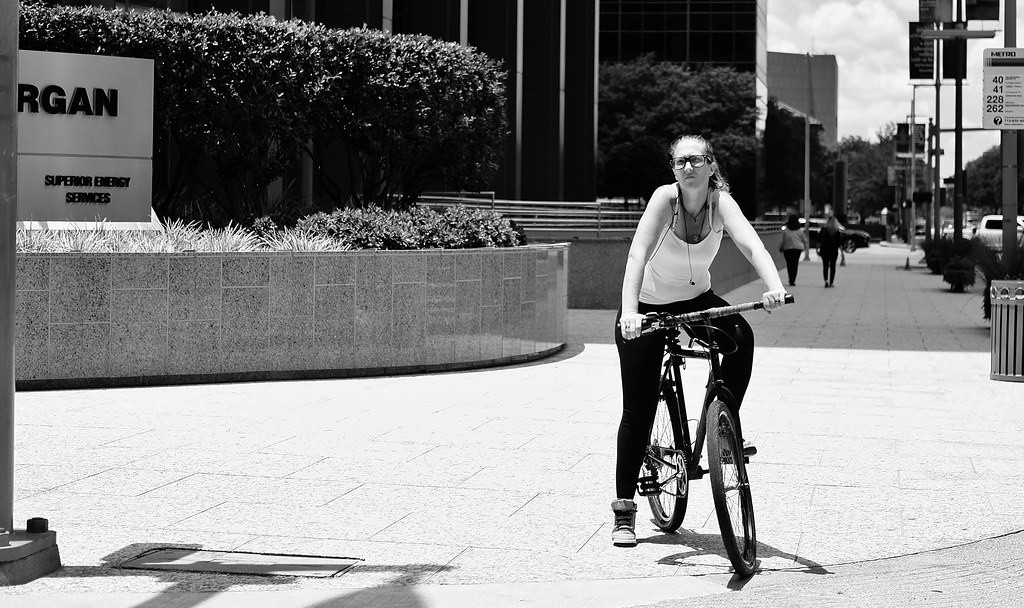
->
[914,213,1024,261]
[781,216,872,254]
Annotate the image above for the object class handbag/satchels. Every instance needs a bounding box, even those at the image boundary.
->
[840,256,846,266]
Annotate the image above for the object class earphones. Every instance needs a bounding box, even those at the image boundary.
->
[689,279,695,285]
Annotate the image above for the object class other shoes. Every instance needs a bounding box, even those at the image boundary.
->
[825,282,835,287]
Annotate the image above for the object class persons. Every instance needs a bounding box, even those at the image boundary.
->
[612,136,787,548]
[817,216,841,288]
[779,213,807,286]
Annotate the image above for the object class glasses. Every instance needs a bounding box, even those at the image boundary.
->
[669,155,713,170]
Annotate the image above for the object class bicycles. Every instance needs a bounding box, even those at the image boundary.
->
[614,294,796,578]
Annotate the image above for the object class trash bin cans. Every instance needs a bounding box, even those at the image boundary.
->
[991,278,1024,382]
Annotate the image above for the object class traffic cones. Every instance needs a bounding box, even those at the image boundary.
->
[903,257,912,270]
[840,255,846,267]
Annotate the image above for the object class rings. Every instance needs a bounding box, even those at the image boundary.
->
[625,326,630,328]
[775,300,780,303]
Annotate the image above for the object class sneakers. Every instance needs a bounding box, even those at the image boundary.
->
[720,431,757,456]
[611,499,637,546]
